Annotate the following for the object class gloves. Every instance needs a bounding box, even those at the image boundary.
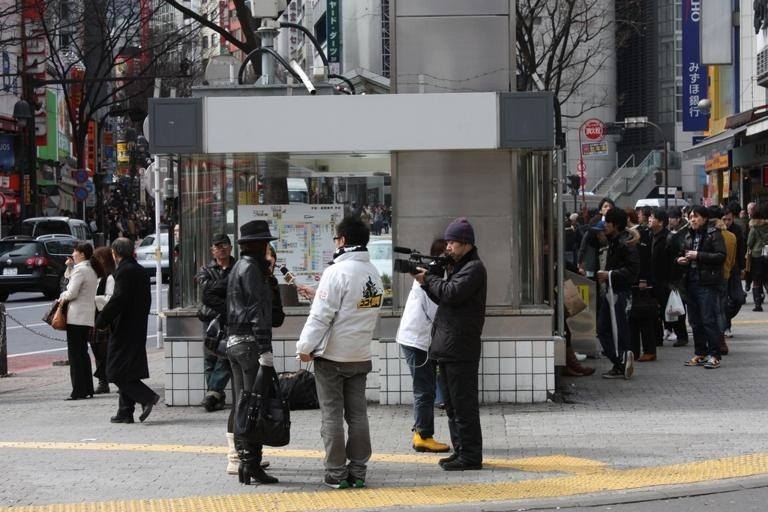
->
[258,353,275,368]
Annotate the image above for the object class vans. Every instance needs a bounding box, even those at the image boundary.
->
[19,215,96,252]
[631,197,690,212]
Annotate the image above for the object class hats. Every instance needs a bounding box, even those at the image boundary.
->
[212,233,229,245]
[234,220,281,245]
[444,216,475,244]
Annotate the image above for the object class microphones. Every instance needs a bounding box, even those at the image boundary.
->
[280,266,309,300]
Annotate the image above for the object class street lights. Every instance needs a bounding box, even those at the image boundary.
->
[93,106,143,248]
[12,89,37,220]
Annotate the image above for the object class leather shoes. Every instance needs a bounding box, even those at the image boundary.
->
[110,412,134,425]
[140,393,160,422]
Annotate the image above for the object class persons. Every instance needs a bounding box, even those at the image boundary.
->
[395,240,451,453]
[351,201,393,236]
[202,244,286,476]
[198,232,238,412]
[411,216,487,472]
[225,220,292,485]
[296,217,387,488]
[549,197,768,380]
[41,179,160,423]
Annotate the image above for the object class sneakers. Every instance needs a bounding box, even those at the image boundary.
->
[348,472,367,487]
[201,394,227,411]
[437,456,482,471]
[412,431,449,451]
[94,381,110,393]
[322,474,348,489]
[568,328,734,380]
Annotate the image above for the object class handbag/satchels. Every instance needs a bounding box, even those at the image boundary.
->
[42,300,67,330]
[233,389,293,447]
[554,278,588,315]
[278,370,320,411]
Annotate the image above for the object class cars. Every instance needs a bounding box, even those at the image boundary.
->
[132,229,170,284]
[224,210,235,258]
[365,238,392,282]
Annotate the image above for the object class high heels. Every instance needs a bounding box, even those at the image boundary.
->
[238,449,278,484]
[66,389,94,400]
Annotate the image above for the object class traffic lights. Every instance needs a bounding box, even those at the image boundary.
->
[653,172,662,186]
[566,175,579,190]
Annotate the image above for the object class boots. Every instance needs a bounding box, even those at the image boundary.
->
[751,288,763,312]
[227,432,240,475]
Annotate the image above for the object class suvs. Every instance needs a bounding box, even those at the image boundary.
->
[0,232,86,301]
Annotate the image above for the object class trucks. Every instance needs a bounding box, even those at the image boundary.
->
[286,177,312,205]
